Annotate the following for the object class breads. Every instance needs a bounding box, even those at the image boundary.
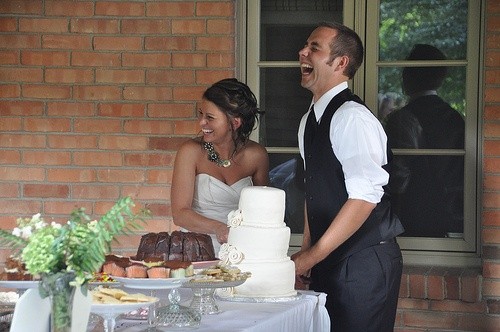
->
[0,258,33,281]
[90,288,156,304]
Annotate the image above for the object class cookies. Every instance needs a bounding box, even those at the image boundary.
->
[190,264,242,282]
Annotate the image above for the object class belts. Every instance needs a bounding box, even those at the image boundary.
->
[379,237,396,244]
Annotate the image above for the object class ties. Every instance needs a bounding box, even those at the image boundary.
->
[304,104,316,171]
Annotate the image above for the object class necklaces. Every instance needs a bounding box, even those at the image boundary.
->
[204,142,231,168]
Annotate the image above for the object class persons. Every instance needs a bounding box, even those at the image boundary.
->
[386,43,464,238]
[290,22,404,332]
[170,79,270,259]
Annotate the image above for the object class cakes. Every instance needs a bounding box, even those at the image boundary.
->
[214,186,297,297]
[134,231,215,261]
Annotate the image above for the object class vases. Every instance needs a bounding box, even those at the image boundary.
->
[40,272,76,332]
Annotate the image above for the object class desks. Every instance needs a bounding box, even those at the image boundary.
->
[87,289,331,332]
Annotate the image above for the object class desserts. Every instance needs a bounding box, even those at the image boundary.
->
[101,254,194,279]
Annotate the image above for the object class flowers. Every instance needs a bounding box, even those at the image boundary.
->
[0,194,153,326]
